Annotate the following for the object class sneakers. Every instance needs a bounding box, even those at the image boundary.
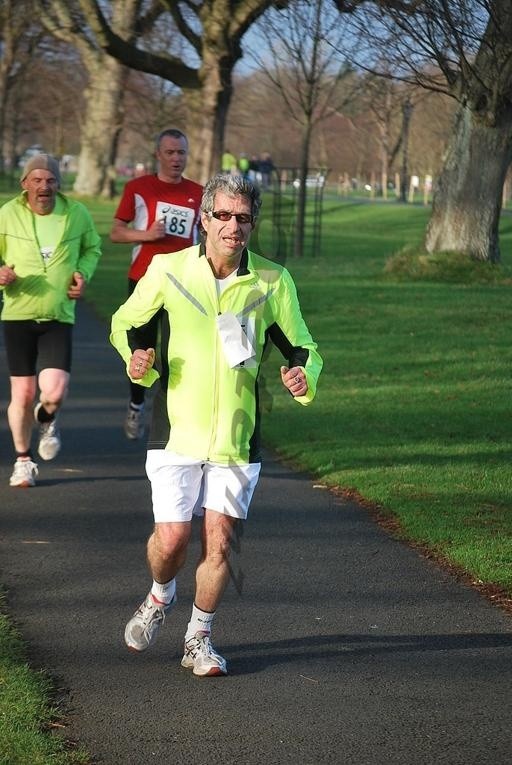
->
[181,630,228,677]
[9,457,39,486]
[123,396,147,440]
[33,402,61,461]
[124,593,177,653]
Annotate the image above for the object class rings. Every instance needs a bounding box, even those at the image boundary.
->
[137,359,143,365]
[135,365,141,373]
[293,377,300,384]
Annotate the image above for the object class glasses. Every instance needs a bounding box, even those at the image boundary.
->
[202,209,255,224]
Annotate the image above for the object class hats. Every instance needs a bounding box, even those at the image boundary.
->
[20,153,62,190]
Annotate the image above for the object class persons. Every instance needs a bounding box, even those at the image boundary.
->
[222,149,273,187]
[0,151,104,488]
[109,128,206,442]
[110,171,324,678]
